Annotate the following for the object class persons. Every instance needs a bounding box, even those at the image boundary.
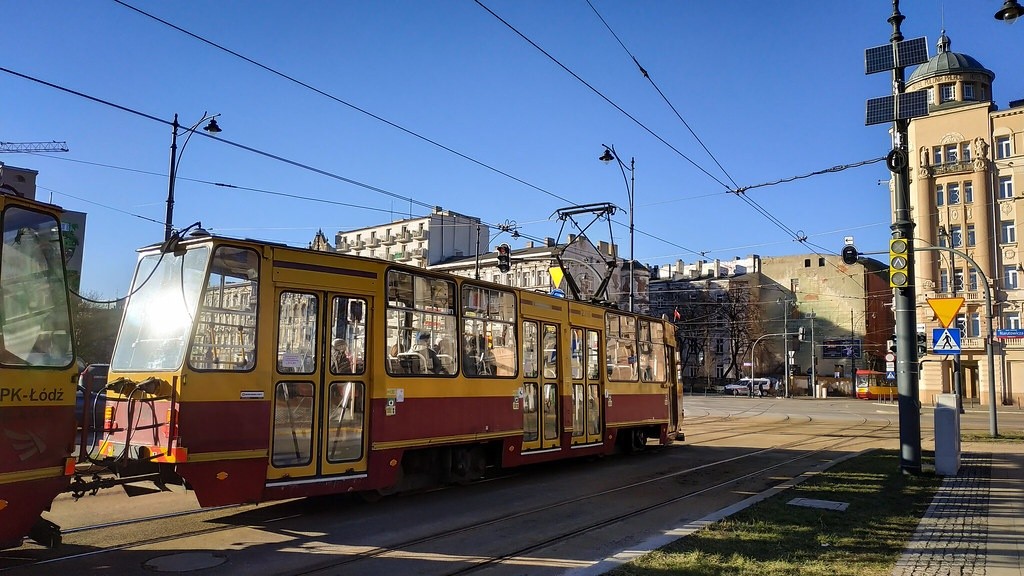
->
[464,336,498,375]
[388,344,404,358]
[775,381,784,397]
[759,382,764,398]
[408,330,441,374]
[331,339,351,373]
[747,380,752,398]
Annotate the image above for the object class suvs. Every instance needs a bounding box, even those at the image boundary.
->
[724,377,770,397]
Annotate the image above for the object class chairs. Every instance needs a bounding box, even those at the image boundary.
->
[542,360,633,381]
[241,339,515,376]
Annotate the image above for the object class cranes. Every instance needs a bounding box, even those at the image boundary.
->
[0,141,72,187]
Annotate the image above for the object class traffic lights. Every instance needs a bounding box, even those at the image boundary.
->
[916,332,927,356]
[890,334,897,356]
[496,243,511,273]
[889,239,907,287]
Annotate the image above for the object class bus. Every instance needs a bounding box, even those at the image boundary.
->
[0,191,693,559]
[856,369,899,401]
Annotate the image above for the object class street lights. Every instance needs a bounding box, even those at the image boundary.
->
[475,218,523,283]
[851,312,878,399]
[805,313,818,399]
[600,142,638,314]
[163,109,223,241]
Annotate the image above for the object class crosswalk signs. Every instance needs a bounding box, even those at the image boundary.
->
[887,372,895,380]
[933,328,961,354]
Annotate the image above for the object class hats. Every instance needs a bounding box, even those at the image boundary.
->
[415,331,429,339]
